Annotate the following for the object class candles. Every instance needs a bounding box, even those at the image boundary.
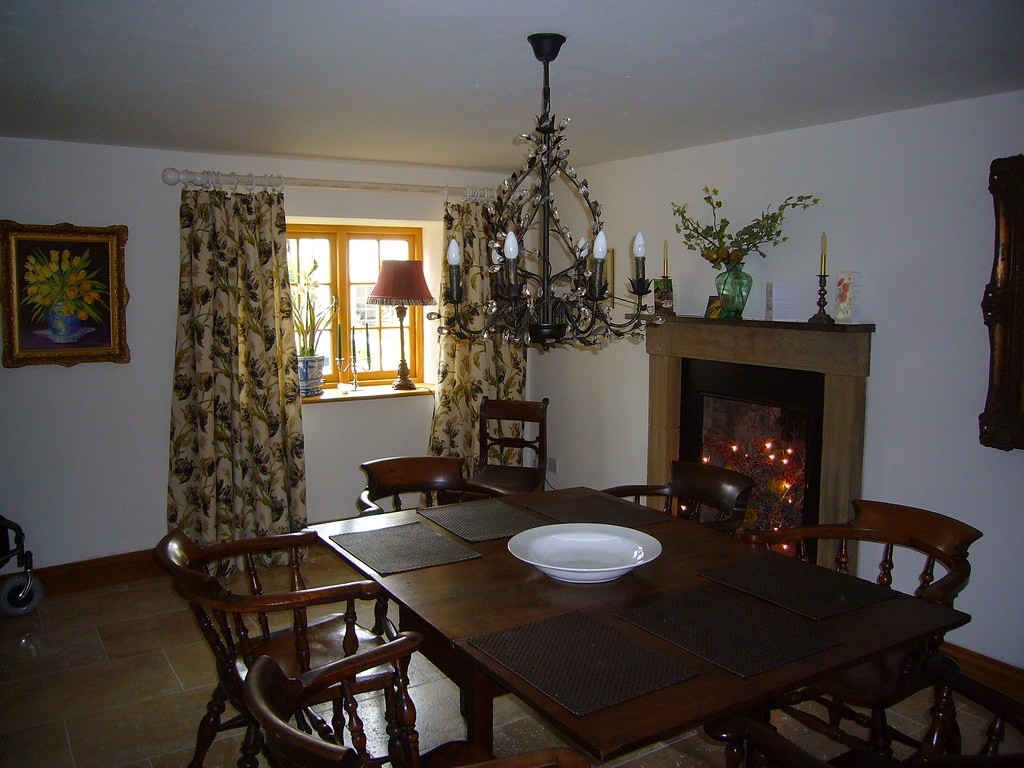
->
[821,232,826,275]
[366,323,370,357]
[663,240,668,276]
[352,326,356,356]
[338,324,342,359]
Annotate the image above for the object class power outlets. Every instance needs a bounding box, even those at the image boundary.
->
[533,456,538,467]
[547,457,557,473]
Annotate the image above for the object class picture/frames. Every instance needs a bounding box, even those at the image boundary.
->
[580,249,615,309]
[0,218,131,369]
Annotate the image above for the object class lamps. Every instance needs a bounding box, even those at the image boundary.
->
[427,32,674,351]
[367,260,436,391]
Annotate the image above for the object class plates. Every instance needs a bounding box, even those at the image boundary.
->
[508,524,662,582]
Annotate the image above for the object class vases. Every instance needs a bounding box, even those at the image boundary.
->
[715,262,753,320]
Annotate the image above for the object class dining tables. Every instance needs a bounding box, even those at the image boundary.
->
[302,485,972,763]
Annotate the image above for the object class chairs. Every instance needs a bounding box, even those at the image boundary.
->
[446,396,549,503]
[355,455,506,635]
[601,460,758,539]
[725,654,1024,768]
[154,528,397,768]
[241,632,590,768]
[743,499,985,752]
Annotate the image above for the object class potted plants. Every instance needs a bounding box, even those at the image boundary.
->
[290,258,341,398]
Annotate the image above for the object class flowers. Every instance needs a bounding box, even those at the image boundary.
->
[671,185,821,306]
